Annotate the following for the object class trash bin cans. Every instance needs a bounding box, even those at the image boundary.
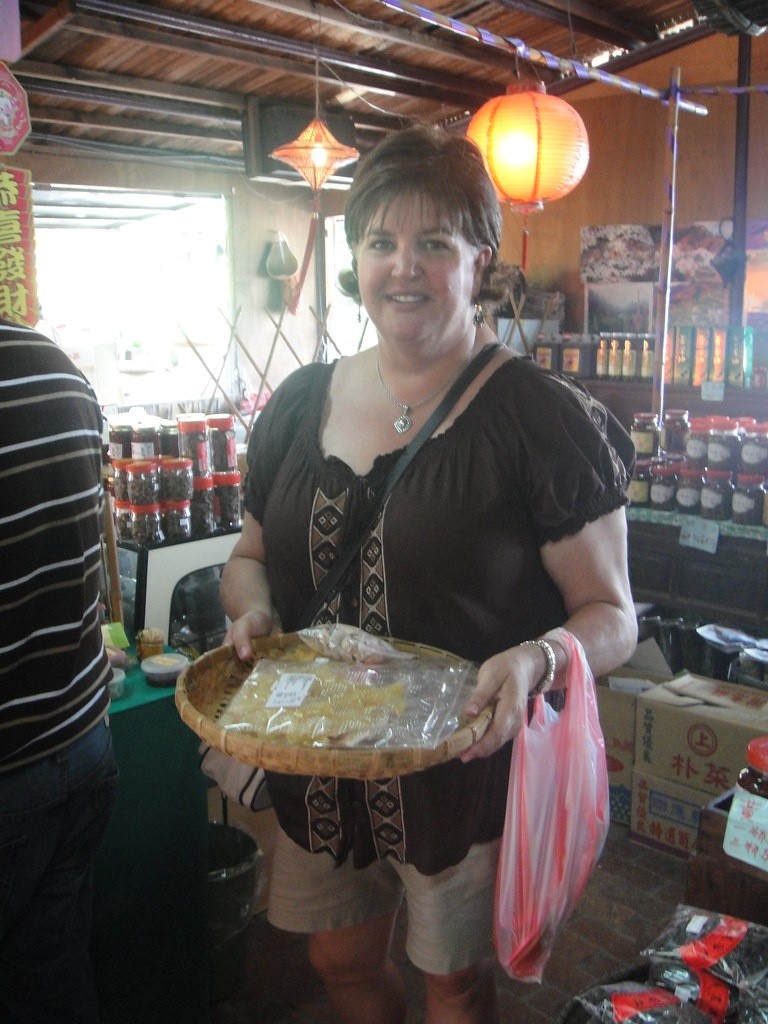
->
[204,822,262,1005]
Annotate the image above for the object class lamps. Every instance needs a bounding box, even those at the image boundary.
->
[265,5,362,187]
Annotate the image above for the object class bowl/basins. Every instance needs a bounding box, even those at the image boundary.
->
[107,667,126,700]
[141,652,189,684]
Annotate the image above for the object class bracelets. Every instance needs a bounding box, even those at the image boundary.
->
[521,639,556,700]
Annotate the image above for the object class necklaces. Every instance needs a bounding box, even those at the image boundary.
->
[375,329,479,436]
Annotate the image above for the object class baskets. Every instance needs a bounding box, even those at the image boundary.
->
[173,631,494,779]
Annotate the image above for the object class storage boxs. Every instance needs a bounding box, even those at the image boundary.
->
[588,667,768,849]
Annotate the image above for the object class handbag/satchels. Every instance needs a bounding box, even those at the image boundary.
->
[492,626,610,983]
[195,742,274,813]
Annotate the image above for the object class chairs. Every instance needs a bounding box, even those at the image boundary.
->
[166,562,235,823]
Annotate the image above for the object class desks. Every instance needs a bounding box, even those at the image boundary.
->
[621,508,767,640]
[576,377,768,429]
[115,526,248,644]
[99,638,209,908]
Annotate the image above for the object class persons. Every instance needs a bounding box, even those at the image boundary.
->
[0,318,121,1024]
[219,124,637,1024]
